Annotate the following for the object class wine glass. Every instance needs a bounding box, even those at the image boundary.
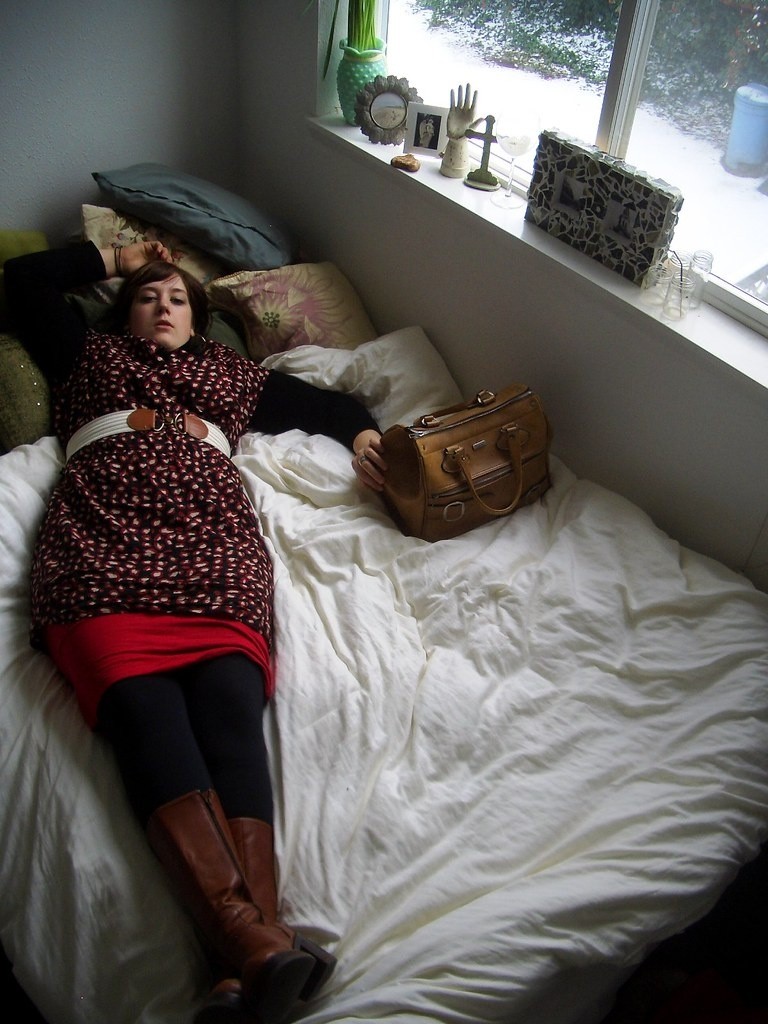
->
[490,98,542,210]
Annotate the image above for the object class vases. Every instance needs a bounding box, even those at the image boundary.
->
[336,36,387,127]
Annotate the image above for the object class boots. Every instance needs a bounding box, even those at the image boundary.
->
[141,787,337,1024]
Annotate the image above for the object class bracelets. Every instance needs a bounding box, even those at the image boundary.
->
[114,245,123,275]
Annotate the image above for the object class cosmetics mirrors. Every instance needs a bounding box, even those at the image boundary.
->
[354,75,424,145]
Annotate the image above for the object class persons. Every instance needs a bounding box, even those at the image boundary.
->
[439,83,485,179]
[420,118,434,147]
[0,238,388,1024]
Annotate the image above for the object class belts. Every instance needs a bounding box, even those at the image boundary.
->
[65,408,232,462]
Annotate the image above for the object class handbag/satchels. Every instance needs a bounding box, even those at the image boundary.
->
[376,383,554,542]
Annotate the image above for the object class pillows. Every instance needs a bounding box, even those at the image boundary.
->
[209,309,251,361]
[209,261,380,365]
[83,202,212,288]
[89,162,302,271]
[0,333,51,454]
[0,229,50,276]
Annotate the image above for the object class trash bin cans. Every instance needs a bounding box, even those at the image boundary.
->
[724,83,768,178]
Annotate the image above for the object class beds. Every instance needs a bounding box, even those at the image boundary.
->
[0,235,768,1024]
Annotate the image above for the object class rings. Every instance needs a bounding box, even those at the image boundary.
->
[358,456,366,465]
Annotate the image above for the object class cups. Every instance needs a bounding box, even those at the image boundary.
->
[641,250,714,322]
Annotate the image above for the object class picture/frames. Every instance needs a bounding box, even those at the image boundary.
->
[402,102,450,159]
